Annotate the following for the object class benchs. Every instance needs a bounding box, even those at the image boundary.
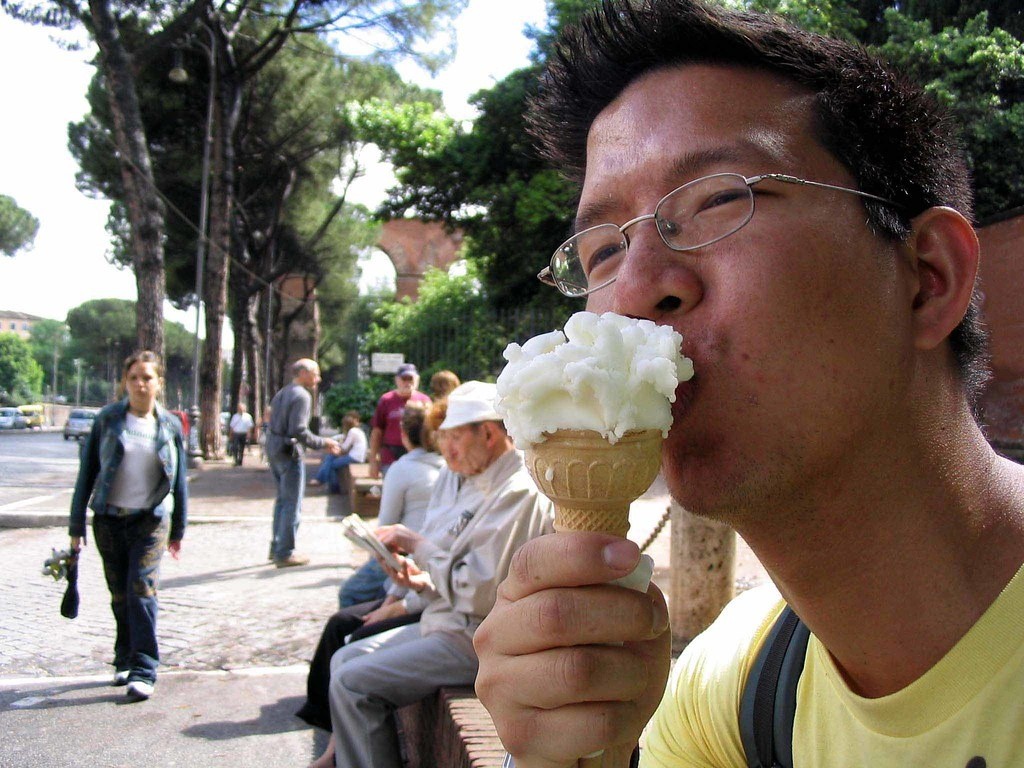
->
[397,685,509,768]
[336,462,384,518]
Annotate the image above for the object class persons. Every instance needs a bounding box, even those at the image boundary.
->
[69,349,187,698]
[227,402,254,467]
[431,370,460,399]
[367,362,430,480]
[257,406,270,464]
[293,380,557,768]
[262,358,345,566]
[471,0,1023,768]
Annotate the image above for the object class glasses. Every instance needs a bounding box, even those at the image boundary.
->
[537,173,891,298]
[399,376,414,382]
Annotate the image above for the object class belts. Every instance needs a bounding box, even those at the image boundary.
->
[104,503,141,515]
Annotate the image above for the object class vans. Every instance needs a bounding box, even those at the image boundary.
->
[17,405,46,428]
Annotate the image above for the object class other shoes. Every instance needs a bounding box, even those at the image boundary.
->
[114,668,131,684]
[269,552,310,568]
[127,681,153,697]
[308,478,322,486]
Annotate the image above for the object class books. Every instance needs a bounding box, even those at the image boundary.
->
[341,511,401,571]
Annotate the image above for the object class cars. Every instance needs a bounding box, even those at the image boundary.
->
[63,409,96,441]
[219,411,231,436]
[0,407,27,430]
[167,410,190,439]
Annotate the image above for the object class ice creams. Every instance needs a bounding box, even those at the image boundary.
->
[497,313,696,541]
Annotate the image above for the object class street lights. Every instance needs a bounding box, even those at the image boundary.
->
[167,38,217,470]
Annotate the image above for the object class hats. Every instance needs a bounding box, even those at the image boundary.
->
[398,363,418,378]
[437,380,503,431]
